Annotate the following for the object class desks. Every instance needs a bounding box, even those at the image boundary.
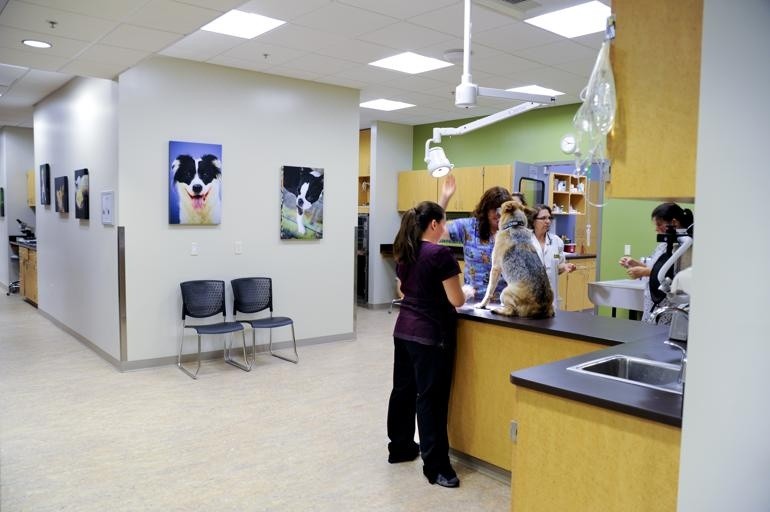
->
[586,278,644,321]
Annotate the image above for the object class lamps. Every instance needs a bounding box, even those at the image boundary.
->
[422,1,557,177]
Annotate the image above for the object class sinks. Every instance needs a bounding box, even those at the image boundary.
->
[566,354,682,396]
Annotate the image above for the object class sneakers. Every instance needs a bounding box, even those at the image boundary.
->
[436,473,461,489]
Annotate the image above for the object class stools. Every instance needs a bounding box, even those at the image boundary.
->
[5,254,19,296]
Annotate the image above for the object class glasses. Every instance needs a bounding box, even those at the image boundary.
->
[535,216,554,219]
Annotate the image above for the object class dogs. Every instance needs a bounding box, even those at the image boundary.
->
[282,166,324,236]
[171,153,221,225]
[473,200,556,320]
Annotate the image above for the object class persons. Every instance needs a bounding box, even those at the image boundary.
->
[618,200,693,326]
[383,201,467,489]
[526,204,576,313]
[512,191,528,213]
[436,170,515,303]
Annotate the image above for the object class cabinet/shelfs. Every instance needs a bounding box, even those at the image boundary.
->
[398,168,437,213]
[607,2,699,202]
[9,235,36,307]
[573,180,596,251]
[548,173,586,215]
[437,160,513,213]
[359,129,370,213]
[555,257,595,312]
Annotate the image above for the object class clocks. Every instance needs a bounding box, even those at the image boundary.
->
[559,133,575,155]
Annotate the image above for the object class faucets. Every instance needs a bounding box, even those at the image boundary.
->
[647,306,689,326]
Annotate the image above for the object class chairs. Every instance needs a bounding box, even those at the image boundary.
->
[229,278,300,369]
[178,279,251,379]
